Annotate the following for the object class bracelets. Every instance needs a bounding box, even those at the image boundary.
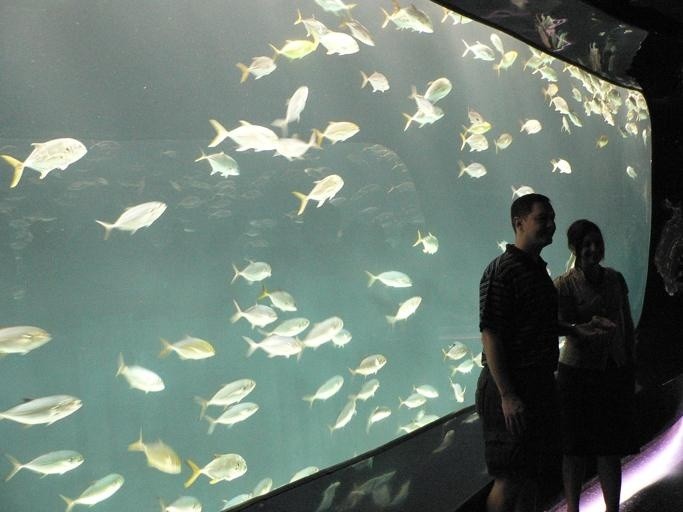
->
[569,322,577,337]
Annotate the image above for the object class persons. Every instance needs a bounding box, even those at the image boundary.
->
[475,194,608,512]
[553,220,637,512]
